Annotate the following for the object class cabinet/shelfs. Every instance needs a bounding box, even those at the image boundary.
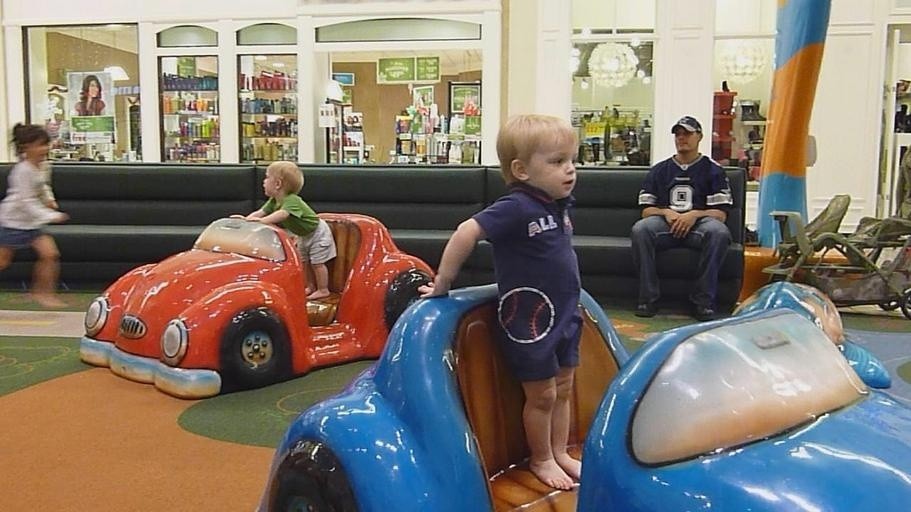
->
[347,138,356,146]
[161,72,220,163]
[238,70,298,161]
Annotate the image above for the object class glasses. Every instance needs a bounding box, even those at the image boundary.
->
[587,29,639,88]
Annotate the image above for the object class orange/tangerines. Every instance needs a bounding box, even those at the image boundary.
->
[764,194,911,317]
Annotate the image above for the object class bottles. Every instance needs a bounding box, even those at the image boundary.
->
[671,116,703,134]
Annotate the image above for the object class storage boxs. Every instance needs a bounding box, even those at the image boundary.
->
[255,164,488,272]
[0,161,254,280]
[476,167,745,311]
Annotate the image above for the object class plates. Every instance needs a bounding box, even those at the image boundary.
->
[161,89,297,164]
[710,92,766,181]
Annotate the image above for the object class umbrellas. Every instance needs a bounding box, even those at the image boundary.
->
[691,304,715,322]
[635,302,659,317]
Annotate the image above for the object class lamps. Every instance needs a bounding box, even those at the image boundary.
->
[455,303,619,512]
[299,220,361,327]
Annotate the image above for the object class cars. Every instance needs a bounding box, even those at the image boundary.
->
[122,151,136,161]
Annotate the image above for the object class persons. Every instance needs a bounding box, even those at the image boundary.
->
[0,122,69,308]
[230,160,338,301]
[418,113,584,492]
[631,116,733,322]
[75,76,105,116]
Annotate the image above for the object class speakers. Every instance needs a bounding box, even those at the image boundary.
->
[741,104,766,121]
